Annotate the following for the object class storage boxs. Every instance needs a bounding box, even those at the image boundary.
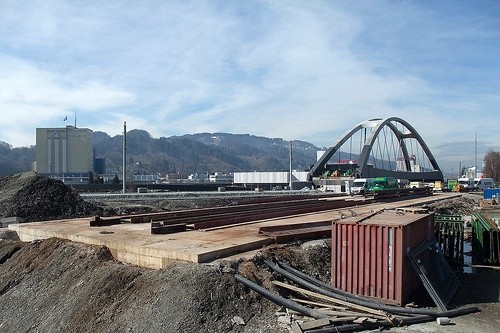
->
[331,209,435,306]
[483,189,500,199]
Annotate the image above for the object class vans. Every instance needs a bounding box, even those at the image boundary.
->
[352,178,374,195]
[370,176,397,195]
[457,178,496,192]
